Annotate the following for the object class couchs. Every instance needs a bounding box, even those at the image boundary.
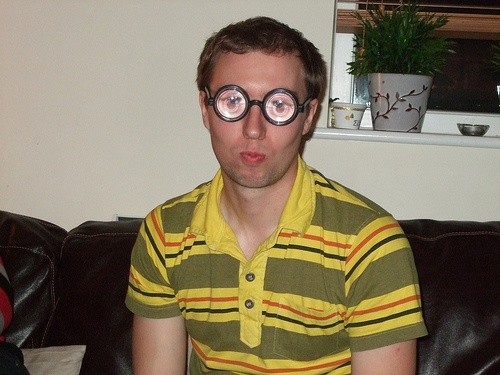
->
[0,210,500,375]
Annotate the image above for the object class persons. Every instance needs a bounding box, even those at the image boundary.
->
[125,17,428,375]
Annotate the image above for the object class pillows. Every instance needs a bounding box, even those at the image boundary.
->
[19,345,86,375]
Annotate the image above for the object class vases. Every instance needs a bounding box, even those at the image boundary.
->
[330,102,367,130]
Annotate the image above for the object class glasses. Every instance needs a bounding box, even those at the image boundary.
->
[205,85,313,126]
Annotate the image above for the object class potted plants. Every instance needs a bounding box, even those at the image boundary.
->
[346,4,459,134]
